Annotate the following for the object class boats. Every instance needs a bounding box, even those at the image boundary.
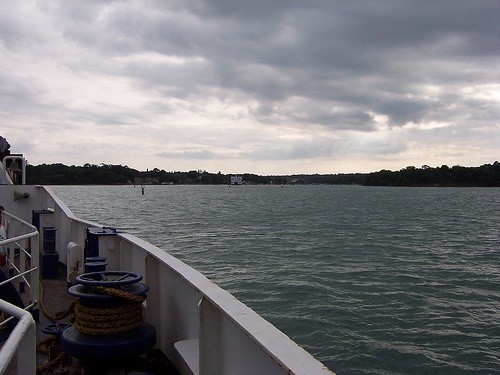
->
[0,133,337,375]
